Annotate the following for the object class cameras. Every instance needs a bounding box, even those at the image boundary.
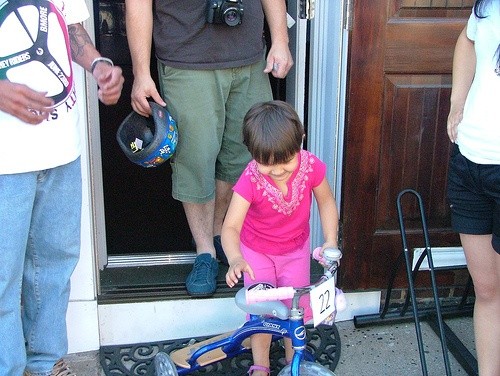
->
[205,0,244,28]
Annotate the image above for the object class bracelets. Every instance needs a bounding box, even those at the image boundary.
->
[91,58,113,73]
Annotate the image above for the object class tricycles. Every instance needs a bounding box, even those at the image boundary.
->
[154,247,343,376]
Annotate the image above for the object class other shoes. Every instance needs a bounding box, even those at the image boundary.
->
[213,236,231,265]
[24,359,75,376]
[186,253,223,297]
[247,366,271,376]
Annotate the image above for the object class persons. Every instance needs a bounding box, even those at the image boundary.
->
[125,1,295,300]
[0,0,125,376]
[446,1,500,376]
[220,99,339,376]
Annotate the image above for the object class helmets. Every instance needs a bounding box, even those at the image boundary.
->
[116,101,179,167]
